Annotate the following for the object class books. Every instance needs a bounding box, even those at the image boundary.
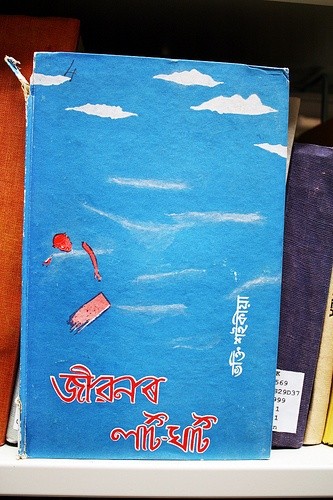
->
[2,48,333,464]
